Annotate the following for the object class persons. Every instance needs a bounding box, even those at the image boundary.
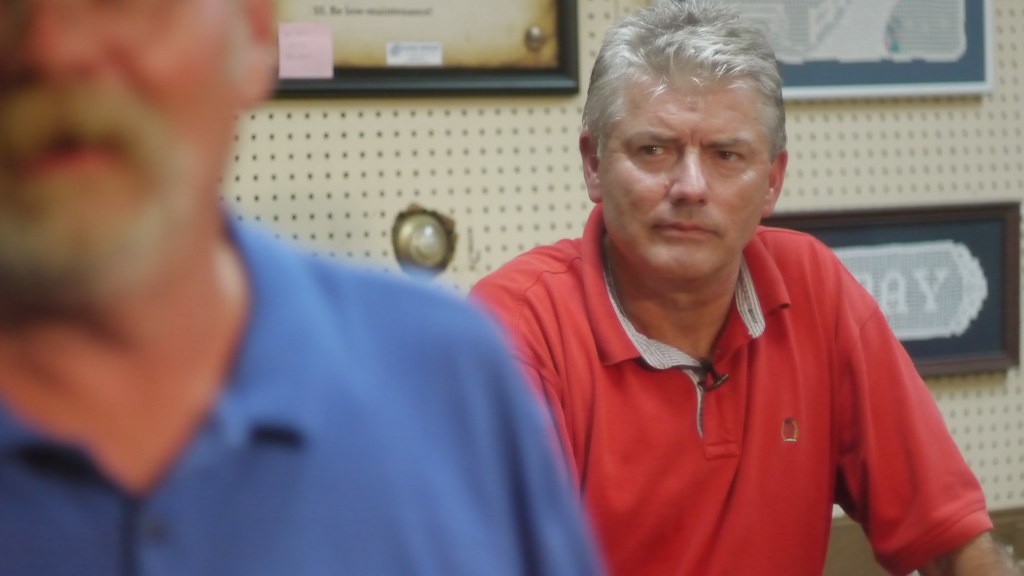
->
[466,0,1021,575]
[1,0,604,574]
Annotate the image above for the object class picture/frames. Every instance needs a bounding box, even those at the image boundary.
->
[755,199,1021,378]
[689,1,997,104]
[270,0,587,94]
